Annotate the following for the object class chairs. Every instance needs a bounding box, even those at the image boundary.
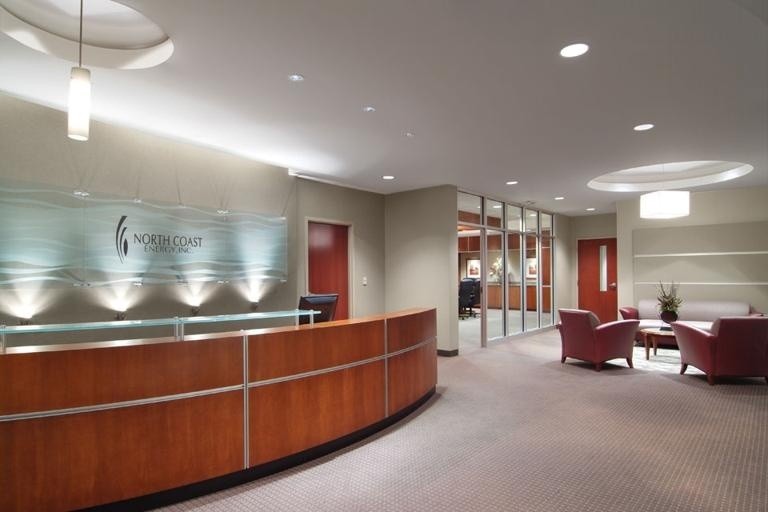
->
[555,309,640,371]
[670,318,768,385]
[458,277,480,320]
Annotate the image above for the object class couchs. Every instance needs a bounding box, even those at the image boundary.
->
[619,300,764,347]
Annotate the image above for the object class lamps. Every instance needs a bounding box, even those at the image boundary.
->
[639,163,690,220]
[67,0,91,142]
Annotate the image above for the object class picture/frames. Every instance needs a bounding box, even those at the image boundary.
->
[466,258,480,278]
[525,258,538,281]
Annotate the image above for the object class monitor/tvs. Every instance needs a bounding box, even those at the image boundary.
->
[298,293,339,325]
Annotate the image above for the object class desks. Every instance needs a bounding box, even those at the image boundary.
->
[640,328,678,359]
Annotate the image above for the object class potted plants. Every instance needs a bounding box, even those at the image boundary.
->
[655,280,685,331]
